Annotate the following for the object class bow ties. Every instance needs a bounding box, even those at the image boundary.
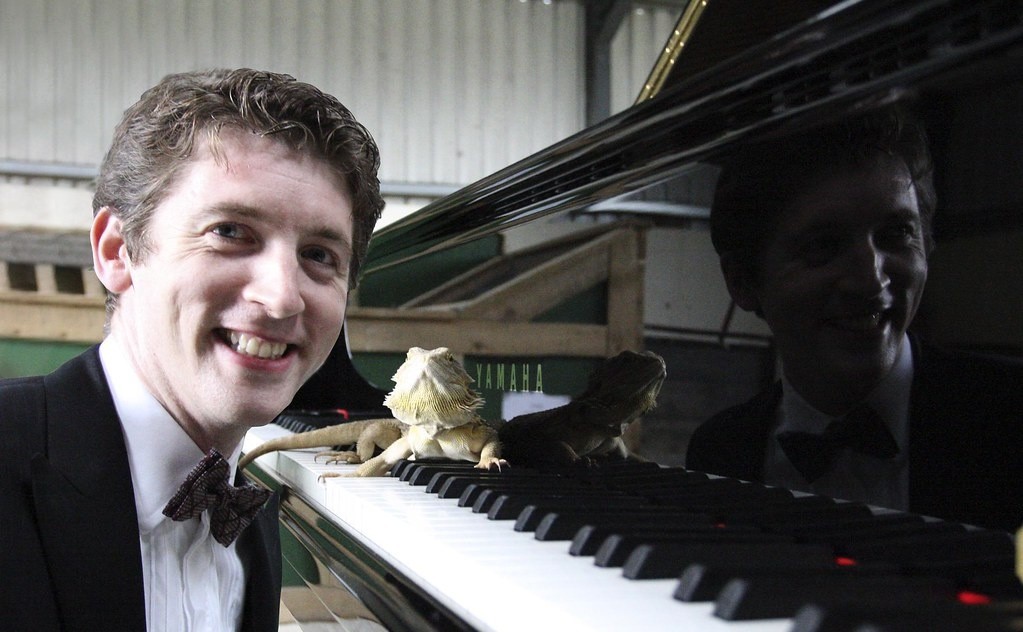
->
[161,449,269,549]
[777,403,902,484]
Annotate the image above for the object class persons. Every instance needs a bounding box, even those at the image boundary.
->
[1,66,385,632]
[685,103,1022,532]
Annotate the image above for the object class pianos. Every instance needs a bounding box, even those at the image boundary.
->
[249,6,1021,632]
